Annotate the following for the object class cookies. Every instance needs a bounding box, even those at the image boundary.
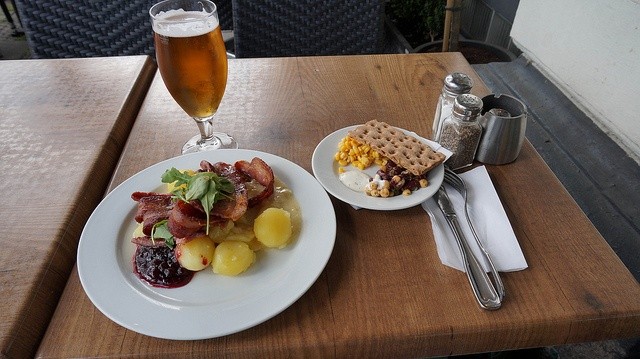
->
[348,116,446,177]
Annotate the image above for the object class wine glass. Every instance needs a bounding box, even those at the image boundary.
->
[148,0,239,156]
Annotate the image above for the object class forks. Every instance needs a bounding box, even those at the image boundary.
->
[444,168,507,303]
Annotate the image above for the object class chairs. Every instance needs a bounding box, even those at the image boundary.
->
[232,0,383,55]
[14,1,235,59]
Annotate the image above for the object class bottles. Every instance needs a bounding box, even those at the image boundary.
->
[432,73,474,143]
[438,94,483,172]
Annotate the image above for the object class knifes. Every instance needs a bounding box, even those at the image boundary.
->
[434,184,502,310]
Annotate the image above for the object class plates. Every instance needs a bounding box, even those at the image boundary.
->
[311,124,445,211]
[77,148,338,341]
